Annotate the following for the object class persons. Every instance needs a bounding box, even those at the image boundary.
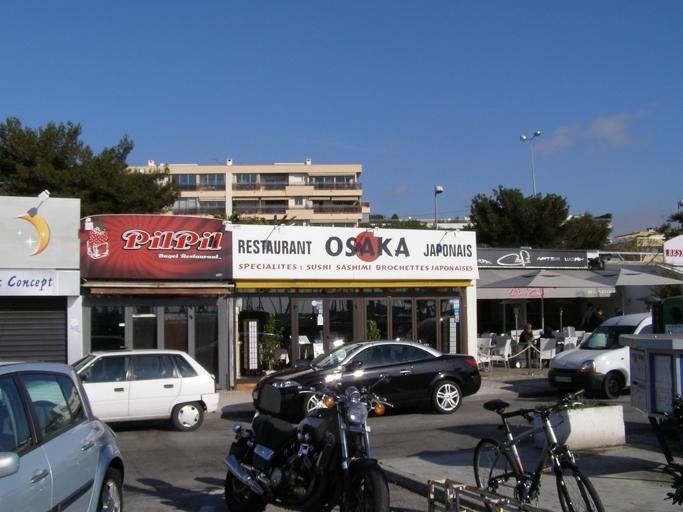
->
[575,302,594,330]
[519,322,537,370]
[534,326,556,368]
[614,306,624,317]
[588,306,606,332]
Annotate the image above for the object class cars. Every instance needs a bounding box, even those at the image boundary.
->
[0,360,124,512]
[70,348,219,432]
[252,339,481,425]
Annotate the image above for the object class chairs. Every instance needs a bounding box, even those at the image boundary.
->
[477,330,586,372]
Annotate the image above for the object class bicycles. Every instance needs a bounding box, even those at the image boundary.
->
[472,389,604,511]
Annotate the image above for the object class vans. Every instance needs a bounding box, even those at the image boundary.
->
[547,311,652,399]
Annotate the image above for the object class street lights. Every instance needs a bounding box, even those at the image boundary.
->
[520,130,540,198]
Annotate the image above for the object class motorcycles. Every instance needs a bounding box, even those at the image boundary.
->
[223,373,394,511]
